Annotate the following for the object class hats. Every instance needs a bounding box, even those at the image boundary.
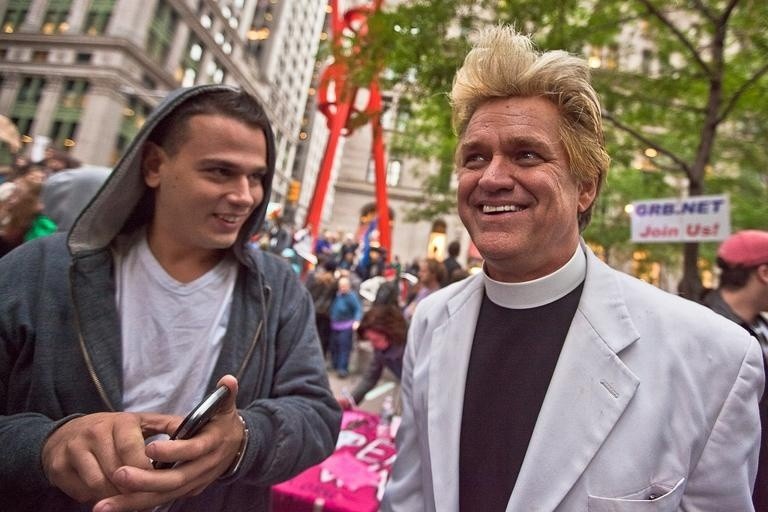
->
[716,230,768,268]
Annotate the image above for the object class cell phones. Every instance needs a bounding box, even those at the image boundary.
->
[152,385,231,469]
